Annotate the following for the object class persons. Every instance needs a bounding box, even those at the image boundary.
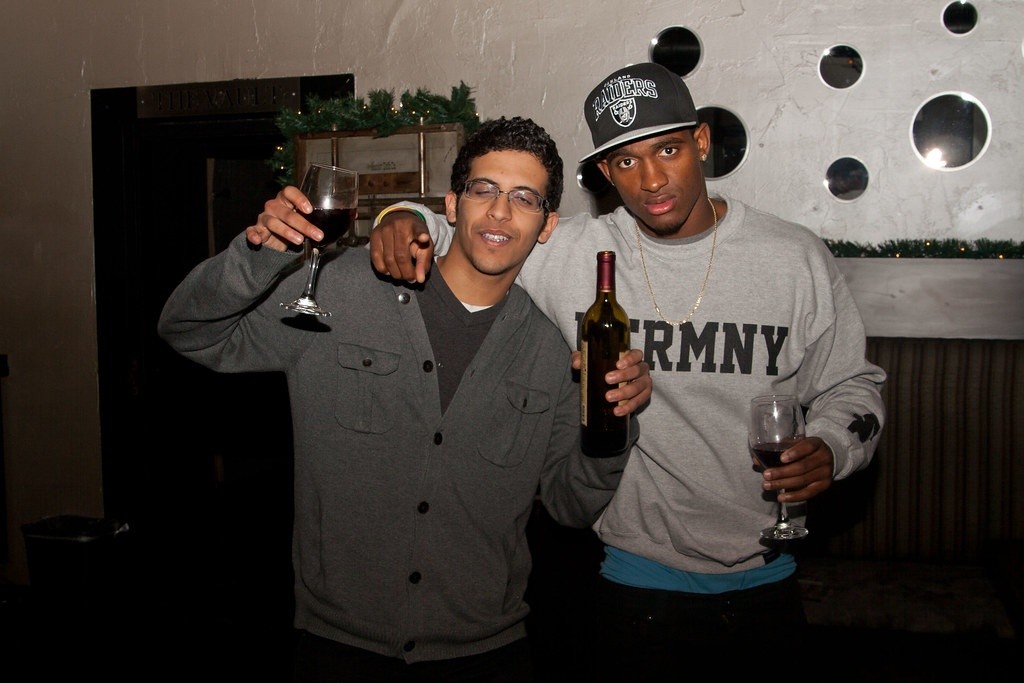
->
[371,63,888,683]
[157,116,653,683]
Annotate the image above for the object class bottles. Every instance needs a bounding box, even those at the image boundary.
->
[581,251,630,458]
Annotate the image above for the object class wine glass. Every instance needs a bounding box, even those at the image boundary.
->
[278,162,358,318]
[748,395,808,539]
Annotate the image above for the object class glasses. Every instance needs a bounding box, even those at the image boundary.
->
[464,181,556,213]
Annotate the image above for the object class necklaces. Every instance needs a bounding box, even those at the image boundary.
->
[634,197,716,326]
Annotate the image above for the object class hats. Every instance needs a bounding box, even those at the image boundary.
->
[578,62,699,163]
